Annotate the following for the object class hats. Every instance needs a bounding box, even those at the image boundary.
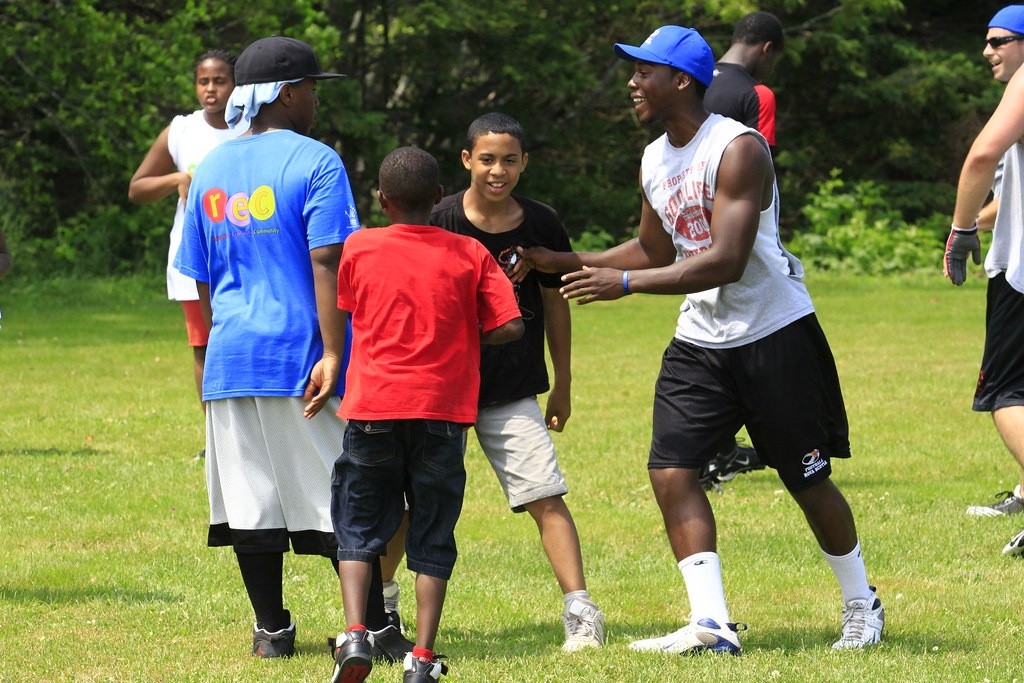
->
[234,35,347,86]
[613,26,715,87]
[989,4,1024,35]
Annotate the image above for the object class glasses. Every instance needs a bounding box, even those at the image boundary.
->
[984,35,1024,48]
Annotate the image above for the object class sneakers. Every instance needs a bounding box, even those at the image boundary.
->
[402,653,448,683]
[252,609,296,658]
[383,580,405,635]
[629,616,746,656]
[966,487,1024,518]
[561,597,604,652]
[368,611,416,662]
[832,587,885,648]
[328,629,375,683]
[700,438,766,481]
[1003,530,1024,557]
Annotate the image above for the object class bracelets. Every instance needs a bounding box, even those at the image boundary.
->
[622,270,632,295]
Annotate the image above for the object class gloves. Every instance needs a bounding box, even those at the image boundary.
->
[944,219,981,287]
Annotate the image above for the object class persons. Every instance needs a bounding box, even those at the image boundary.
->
[128,48,238,458]
[379,113,604,651]
[331,147,525,683]
[944,61,1024,556]
[506,25,885,654]
[966,5,1024,517]
[173,36,448,666]
[696,12,787,491]
